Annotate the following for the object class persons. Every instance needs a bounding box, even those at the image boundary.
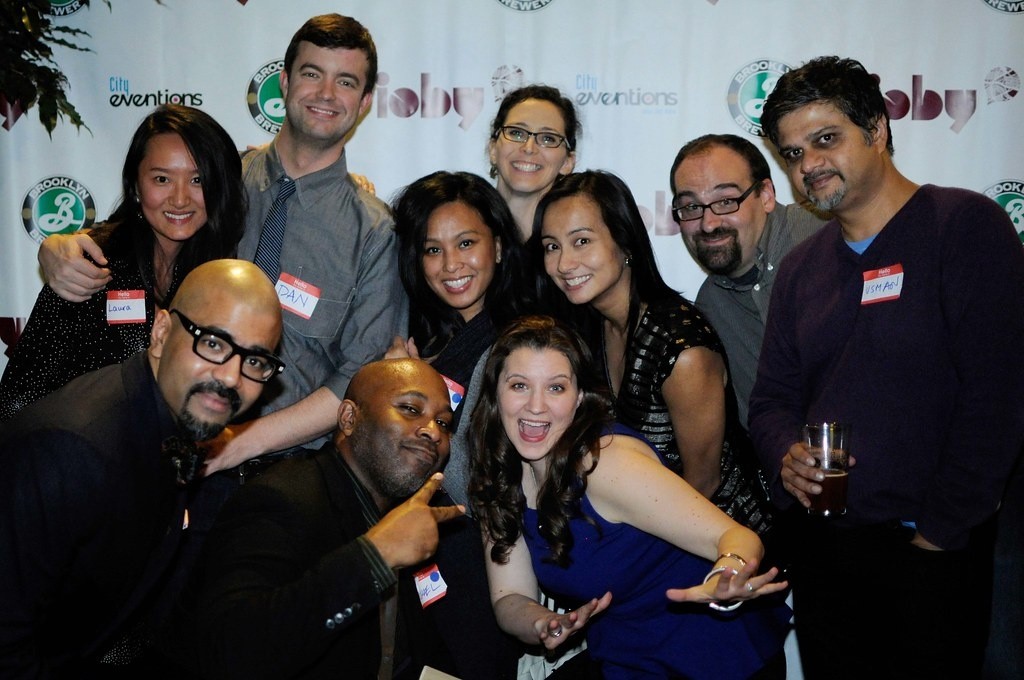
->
[0,13,1024,680]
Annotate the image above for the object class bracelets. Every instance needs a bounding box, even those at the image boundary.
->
[703,553,754,611]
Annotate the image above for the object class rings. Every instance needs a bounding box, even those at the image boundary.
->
[747,583,753,592]
[548,626,563,637]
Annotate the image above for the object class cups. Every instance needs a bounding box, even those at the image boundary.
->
[803,424,849,515]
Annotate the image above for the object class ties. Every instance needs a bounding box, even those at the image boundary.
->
[253,175,296,285]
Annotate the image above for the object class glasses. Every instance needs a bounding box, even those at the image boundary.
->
[494,125,572,151]
[168,308,286,383]
[672,178,767,222]
